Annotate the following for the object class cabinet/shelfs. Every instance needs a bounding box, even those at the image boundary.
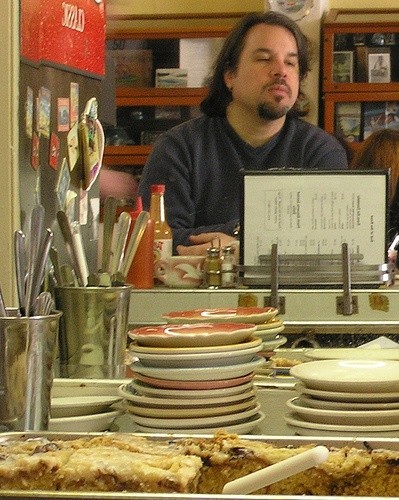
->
[323,4,399,156]
[100,1,321,165]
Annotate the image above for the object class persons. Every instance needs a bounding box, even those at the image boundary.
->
[349,127,399,265]
[133,11,348,257]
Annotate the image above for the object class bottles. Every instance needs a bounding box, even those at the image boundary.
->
[148,184,173,284]
[205,249,222,289]
[221,246,237,287]
[125,197,154,288]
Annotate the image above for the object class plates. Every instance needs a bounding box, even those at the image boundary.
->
[285,347,399,440]
[48,306,288,436]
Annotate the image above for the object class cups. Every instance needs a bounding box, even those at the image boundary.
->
[0,308,64,431]
[156,254,206,289]
[53,274,133,378]
